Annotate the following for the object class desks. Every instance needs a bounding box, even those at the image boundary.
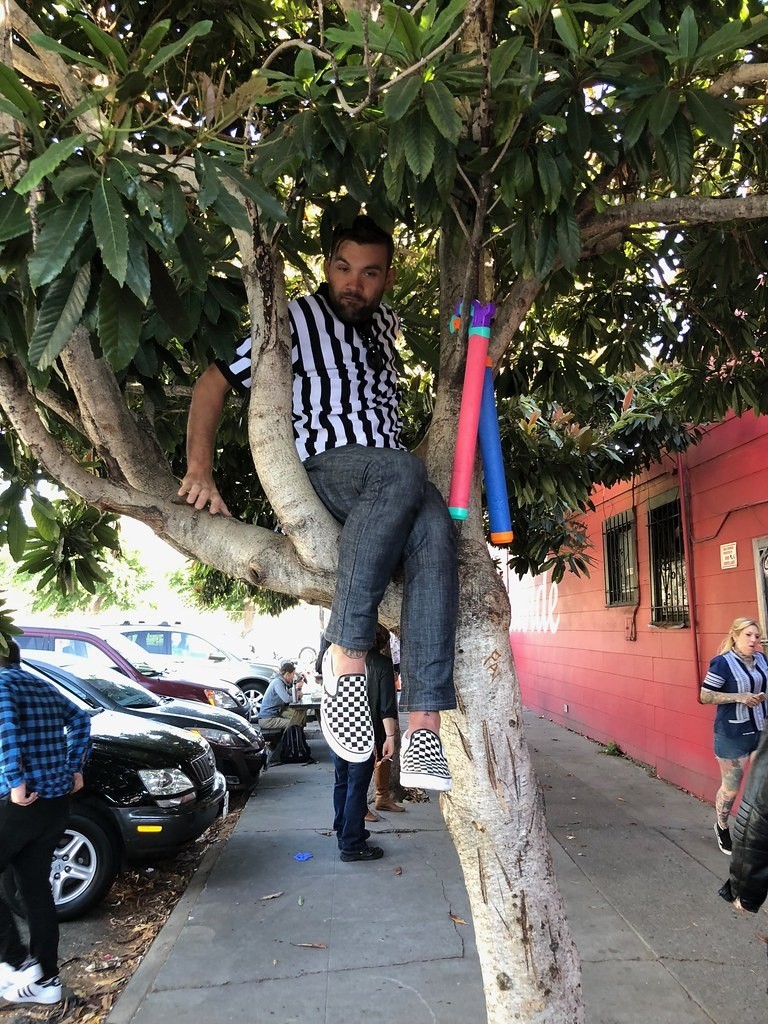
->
[289,694,322,732]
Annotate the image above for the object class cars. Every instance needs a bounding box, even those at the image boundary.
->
[269,633,321,665]
[19,649,272,819]
[67,625,279,724]
[9,622,251,723]
[7,657,230,924]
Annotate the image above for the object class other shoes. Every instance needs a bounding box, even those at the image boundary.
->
[399,726,452,791]
[713,821,733,856]
[340,844,384,862]
[320,644,375,763]
[336,828,370,849]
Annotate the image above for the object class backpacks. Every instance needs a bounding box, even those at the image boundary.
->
[281,724,312,764]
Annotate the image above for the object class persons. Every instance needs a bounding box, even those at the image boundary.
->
[258,662,307,766]
[698,617,765,857]
[718,734,767,915]
[0,623,88,1005]
[174,216,464,792]
[363,676,407,822]
[316,625,398,864]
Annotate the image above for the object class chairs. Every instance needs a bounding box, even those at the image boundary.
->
[63,646,74,654]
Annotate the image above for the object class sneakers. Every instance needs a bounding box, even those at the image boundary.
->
[0,956,44,998]
[3,975,62,1004]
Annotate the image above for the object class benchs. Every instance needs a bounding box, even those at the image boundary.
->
[259,726,281,764]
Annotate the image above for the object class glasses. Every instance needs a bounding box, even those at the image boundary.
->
[355,314,383,371]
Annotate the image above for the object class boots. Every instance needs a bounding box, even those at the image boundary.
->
[374,759,405,812]
[364,809,378,822]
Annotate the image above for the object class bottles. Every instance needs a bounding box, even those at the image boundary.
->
[292,683,298,703]
